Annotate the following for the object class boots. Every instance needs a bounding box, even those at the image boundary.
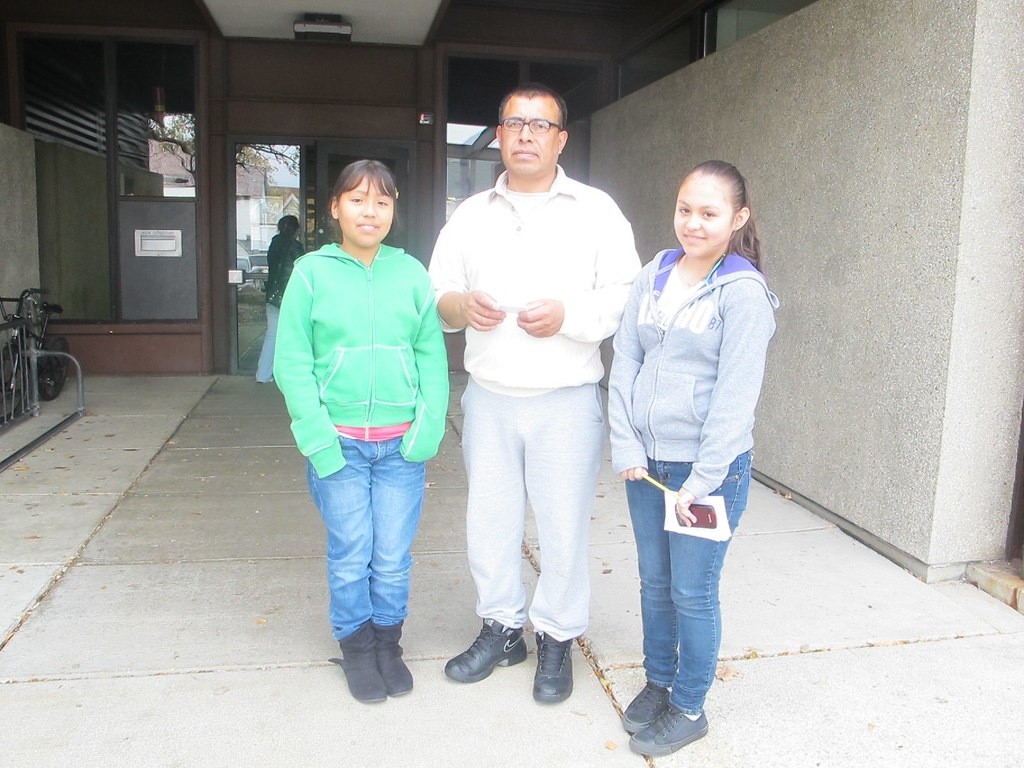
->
[329,619,386,704]
[370,619,414,697]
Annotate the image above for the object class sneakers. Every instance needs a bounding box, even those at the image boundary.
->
[533,632,574,702]
[446,617,526,682]
[623,681,675,733]
[628,704,708,757]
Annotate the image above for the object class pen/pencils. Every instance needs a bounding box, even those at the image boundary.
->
[642,473,674,495]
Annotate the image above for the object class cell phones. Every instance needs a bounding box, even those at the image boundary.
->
[675,503,717,529]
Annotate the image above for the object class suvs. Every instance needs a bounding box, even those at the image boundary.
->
[237,255,268,293]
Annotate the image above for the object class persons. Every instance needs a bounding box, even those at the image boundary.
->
[255,215,306,383]
[272,161,449,702]
[429,84,641,704]
[609,160,775,757]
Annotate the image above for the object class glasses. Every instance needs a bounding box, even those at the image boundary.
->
[499,117,561,135]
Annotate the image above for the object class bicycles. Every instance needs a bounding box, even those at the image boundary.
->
[0,288,69,424]
